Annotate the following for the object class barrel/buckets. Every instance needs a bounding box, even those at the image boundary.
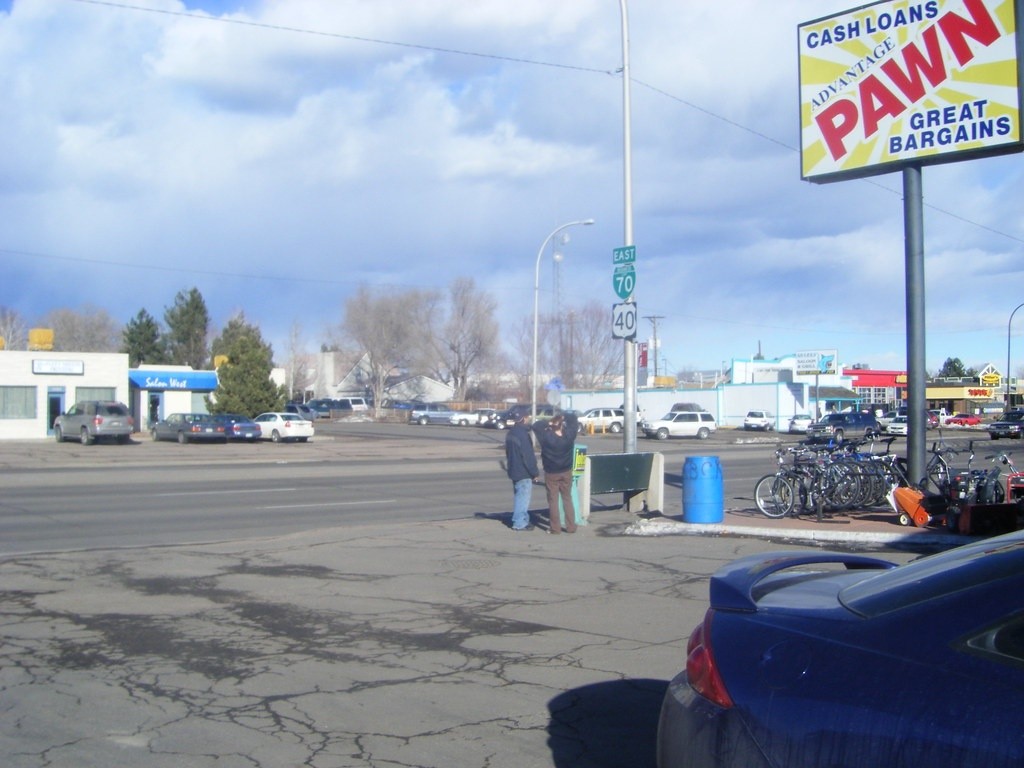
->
[682,456,723,522]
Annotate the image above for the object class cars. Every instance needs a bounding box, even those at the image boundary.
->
[281,396,437,421]
[787,414,814,434]
[945,412,981,426]
[742,409,776,432]
[656,530,1024,768]
[206,414,262,443]
[874,405,954,436]
[447,402,584,431]
[251,412,315,443]
[151,411,226,444]
[619,404,646,427]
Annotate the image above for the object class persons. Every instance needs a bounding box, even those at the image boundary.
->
[505,408,538,531]
[532,412,579,534]
[832,405,836,414]
[868,407,876,417]
[819,408,822,422]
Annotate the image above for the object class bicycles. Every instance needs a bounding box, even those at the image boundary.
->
[753,428,1024,533]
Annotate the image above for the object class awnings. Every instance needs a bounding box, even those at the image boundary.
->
[129,370,217,391]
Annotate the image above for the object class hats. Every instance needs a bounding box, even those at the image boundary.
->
[513,411,527,422]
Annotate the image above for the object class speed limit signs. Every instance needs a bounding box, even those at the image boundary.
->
[610,302,638,341]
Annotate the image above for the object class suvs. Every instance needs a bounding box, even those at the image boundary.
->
[577,407,624,433]
[640,410,718,441]
[988,411,1024,441]
[668,402,706,419]
[53,400,134,446]
[805,411,881,445]
[410,403,468,426]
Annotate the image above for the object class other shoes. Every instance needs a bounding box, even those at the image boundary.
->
[511,526,534,531]
[546,529,551,534]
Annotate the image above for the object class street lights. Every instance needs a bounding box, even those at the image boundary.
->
[529,217,598,448]
